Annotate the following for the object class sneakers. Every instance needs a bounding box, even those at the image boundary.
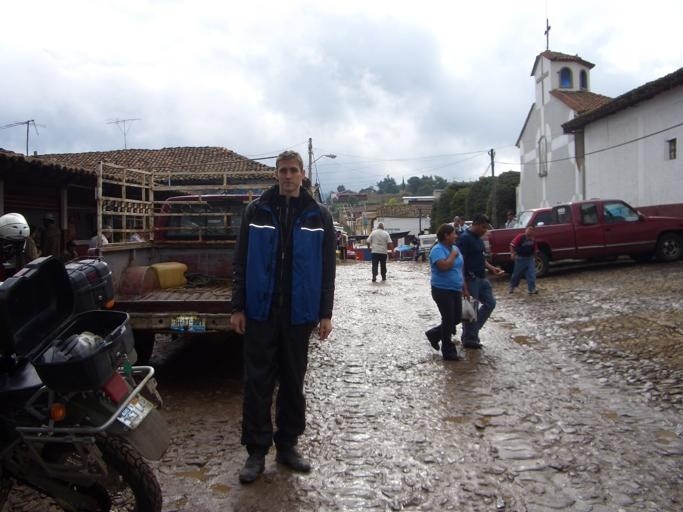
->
[274,443,313,475]
[425,330,440,351]
[238,452,265,485]
[461,340,483,350]
[527,289,540,295]
[506,286,514,294]
[442,353,460,362]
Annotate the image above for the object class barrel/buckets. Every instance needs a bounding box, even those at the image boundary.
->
[151,260,188,288]
[355,244,372,261]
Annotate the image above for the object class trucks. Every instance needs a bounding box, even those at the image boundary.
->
[80,159,318,373]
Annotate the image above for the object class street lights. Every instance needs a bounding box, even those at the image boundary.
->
[307,137,335,192]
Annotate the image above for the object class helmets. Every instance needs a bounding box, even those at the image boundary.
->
[0,211,32,243]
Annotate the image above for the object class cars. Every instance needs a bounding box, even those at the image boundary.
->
[412,200,682,279]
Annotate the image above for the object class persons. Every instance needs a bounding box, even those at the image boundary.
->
[0,212,146,282]
[365,223,395,281]
[228,150,336,485]
[415,210,538,362]
[337,231,348,260]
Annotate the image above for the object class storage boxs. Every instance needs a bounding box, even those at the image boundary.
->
[61,258,115,311]
[0,250,136,396]
